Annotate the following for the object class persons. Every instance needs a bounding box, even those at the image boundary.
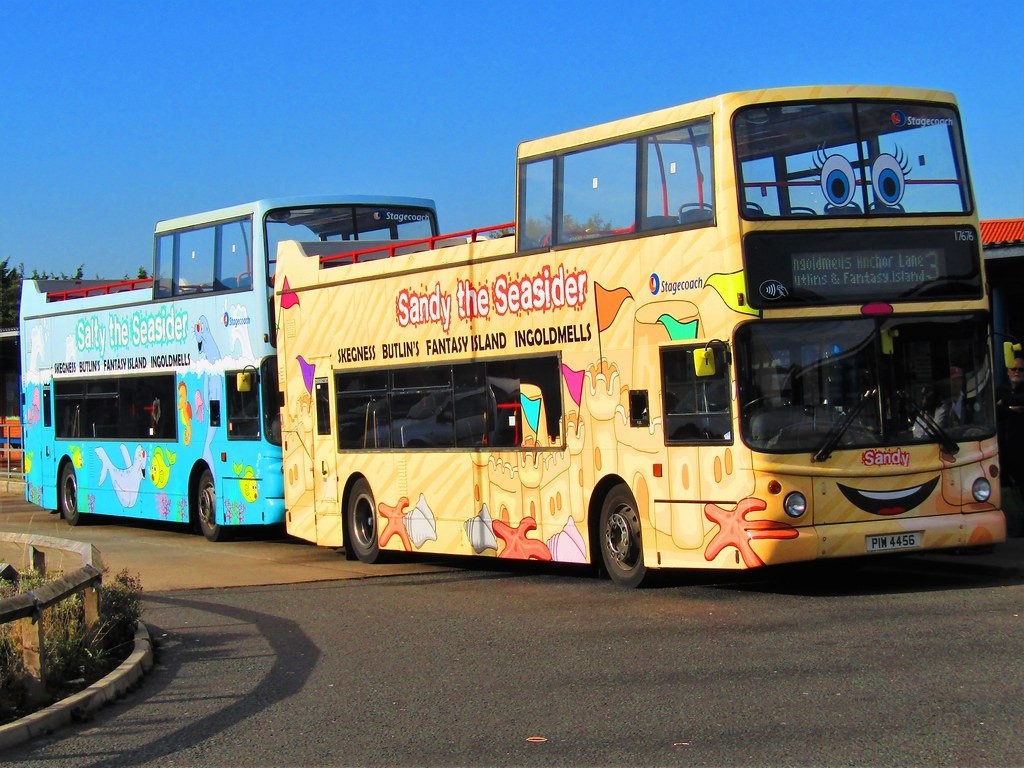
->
[995,359,1024,538]
[912,364,988,438]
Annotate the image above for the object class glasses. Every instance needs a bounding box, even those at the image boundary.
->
[1008,367,1024,372]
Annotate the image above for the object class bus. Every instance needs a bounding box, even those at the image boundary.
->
[273,82,1024,589]
[18,193,444,543]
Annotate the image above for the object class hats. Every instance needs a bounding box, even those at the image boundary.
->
[944,367,962,378]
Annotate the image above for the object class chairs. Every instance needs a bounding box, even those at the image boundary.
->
[647,199,905,230]
[159,271,253,297]
